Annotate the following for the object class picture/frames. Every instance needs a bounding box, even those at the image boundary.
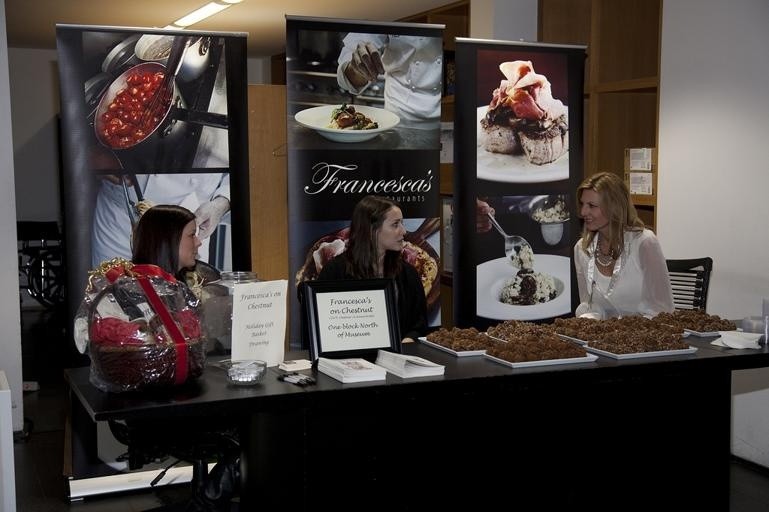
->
[301,280,403,362]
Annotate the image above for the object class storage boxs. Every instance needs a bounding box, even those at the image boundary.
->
[624,148,657,204]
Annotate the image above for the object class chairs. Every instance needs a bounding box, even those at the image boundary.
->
[665,257,712,313]
[187,261,221,284]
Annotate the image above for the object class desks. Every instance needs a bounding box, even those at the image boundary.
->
[62,322,767,511]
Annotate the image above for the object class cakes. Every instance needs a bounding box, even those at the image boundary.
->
[480,60,567,164]
[499,246,556,305]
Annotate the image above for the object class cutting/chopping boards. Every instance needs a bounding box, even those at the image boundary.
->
[295,215,442,322]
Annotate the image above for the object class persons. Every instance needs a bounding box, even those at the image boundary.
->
[475,199,495,234]
[89,174,229,270]
[128,204,200,281]
[297,195,429,344]
[572,170,676,318]
[337,33,443,125]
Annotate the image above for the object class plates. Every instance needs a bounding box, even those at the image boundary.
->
[476,106,568,183]
[417,335,485,357]
[678,330,691,339]
[474,253,577,322]
[582,344,698,361]
[558,333,597,345]
[483,331,511,344]
[293,104,400,143]
[482,351,599,369]
[668,324,745,338]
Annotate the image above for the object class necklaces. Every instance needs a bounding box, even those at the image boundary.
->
[592,243,619,269]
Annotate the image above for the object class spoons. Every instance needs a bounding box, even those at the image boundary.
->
[486,210,533,271]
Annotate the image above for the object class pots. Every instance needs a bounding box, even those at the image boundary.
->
[94,62,228,176]
